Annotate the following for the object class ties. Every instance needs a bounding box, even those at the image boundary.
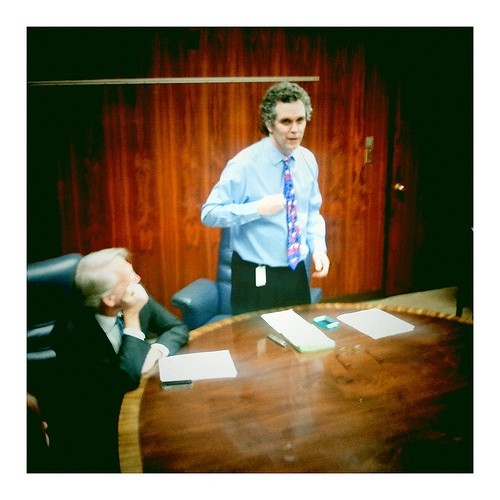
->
[281,158,302,271]
[114,316,126,336]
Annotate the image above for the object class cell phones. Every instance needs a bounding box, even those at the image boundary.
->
[313,315,340,330]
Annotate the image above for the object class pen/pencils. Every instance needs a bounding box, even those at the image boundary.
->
[267,335,287,347]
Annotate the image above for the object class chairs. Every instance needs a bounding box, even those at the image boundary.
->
[171,228,322,331]
[27,253,82,448]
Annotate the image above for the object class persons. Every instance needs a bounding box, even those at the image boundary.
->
[200,83,330,318]
[53,247,189,473]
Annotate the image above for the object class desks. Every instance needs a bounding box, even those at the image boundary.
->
[118,302,474,474]
